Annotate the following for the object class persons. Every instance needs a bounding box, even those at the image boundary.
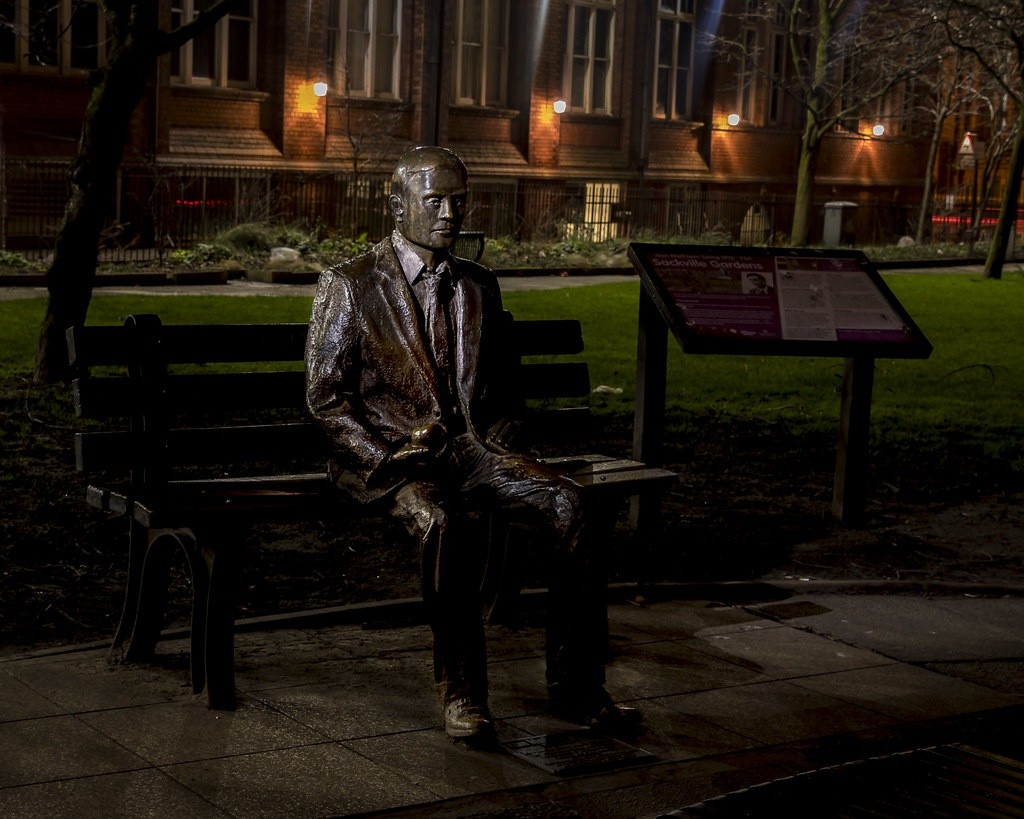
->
[304,146,643,741]
[746,273,774,295]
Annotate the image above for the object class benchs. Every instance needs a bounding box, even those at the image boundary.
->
[63,316,680,715]
